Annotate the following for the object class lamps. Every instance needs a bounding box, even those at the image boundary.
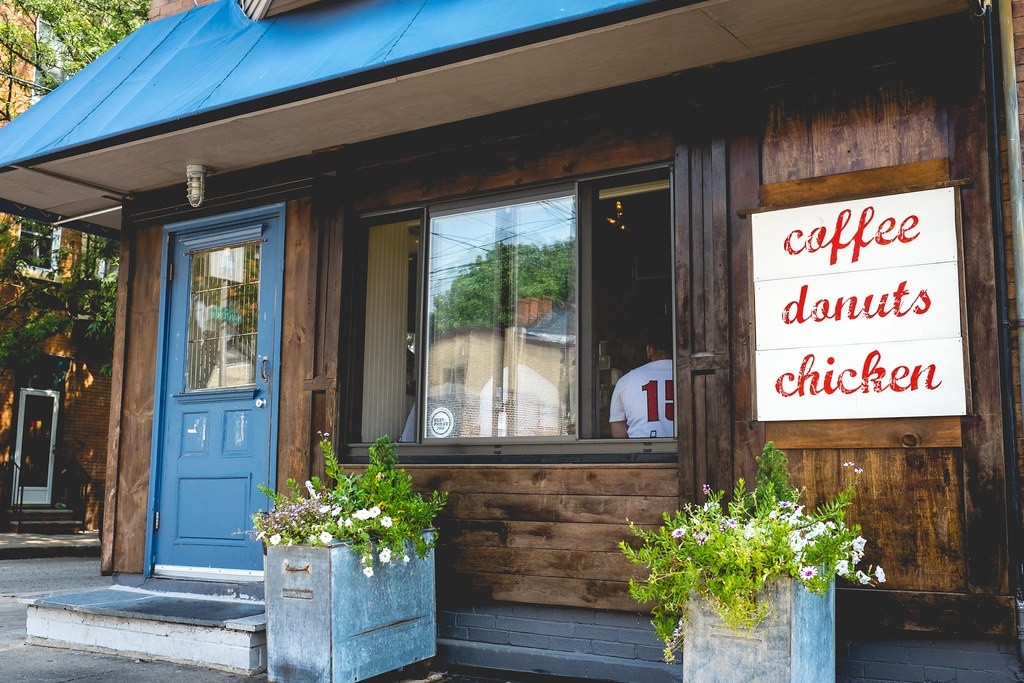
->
[185,164,206,208]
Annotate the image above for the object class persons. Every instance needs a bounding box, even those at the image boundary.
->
[478,345,565,437]
[609,338,675,438]
[398,362,480,443]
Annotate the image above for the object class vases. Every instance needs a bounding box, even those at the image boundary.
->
[682,572,836,683]
[264,528,438,683]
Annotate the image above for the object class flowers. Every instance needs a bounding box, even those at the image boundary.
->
[619,440,886,664]
[241,429,450,579]
